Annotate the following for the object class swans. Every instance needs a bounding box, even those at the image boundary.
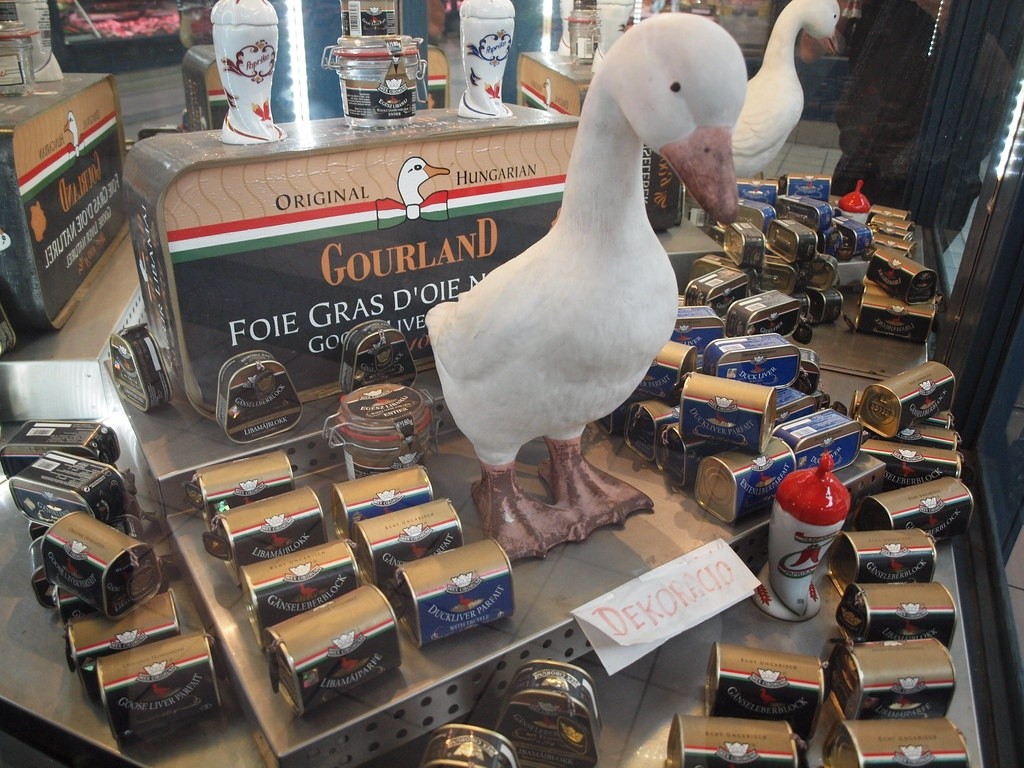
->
[733,1,843,176]
[425,10,749,561]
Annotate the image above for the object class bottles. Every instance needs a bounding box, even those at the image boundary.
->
[321,383,443,473]
[838,179,872,226]
[752,450,852,622]
[319,35,430,128]
[340,0,403,38]
[209,0,288,146]
[598,0,635,55]
[459,0,516,120]
[562,0,601,66]
[0,19,39,96]
